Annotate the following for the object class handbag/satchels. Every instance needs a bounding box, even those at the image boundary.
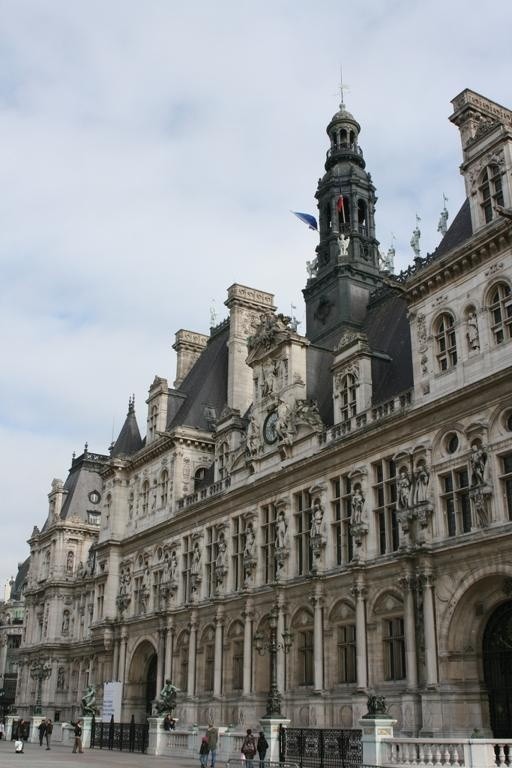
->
[15,741,23,751]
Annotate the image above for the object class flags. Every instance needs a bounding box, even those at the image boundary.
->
[333,193,345,215]
[293,209,320,233]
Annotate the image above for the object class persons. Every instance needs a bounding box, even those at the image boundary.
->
[311,502,322,534]
[242,526,254,558]
[70,719,84,752]
[254,731,270,768]
[466,310,479,347]
[305,260,313,278]
[413,463,429,503]
[407,226,423,256]
[435,205,454,236]
[215,539,226,566]
[204,722,220,767]
[44,718,54,750]
[15,717,25,753]
[160,556,169,582]
[163,713,177,731]
[0,720,6,739]
[241,729,257,768]
[36,718,47,747]
[193,545,201,571]
[467,443,488,489]
[398,471,412,508]
[79,680,96,715]
[196,735,210,768]
[350,488,363,524]
[153,677,182,713]
[385,243,398,267]
[169,555,177,580]
[275,514,287,548]
[244,355,329,448]
